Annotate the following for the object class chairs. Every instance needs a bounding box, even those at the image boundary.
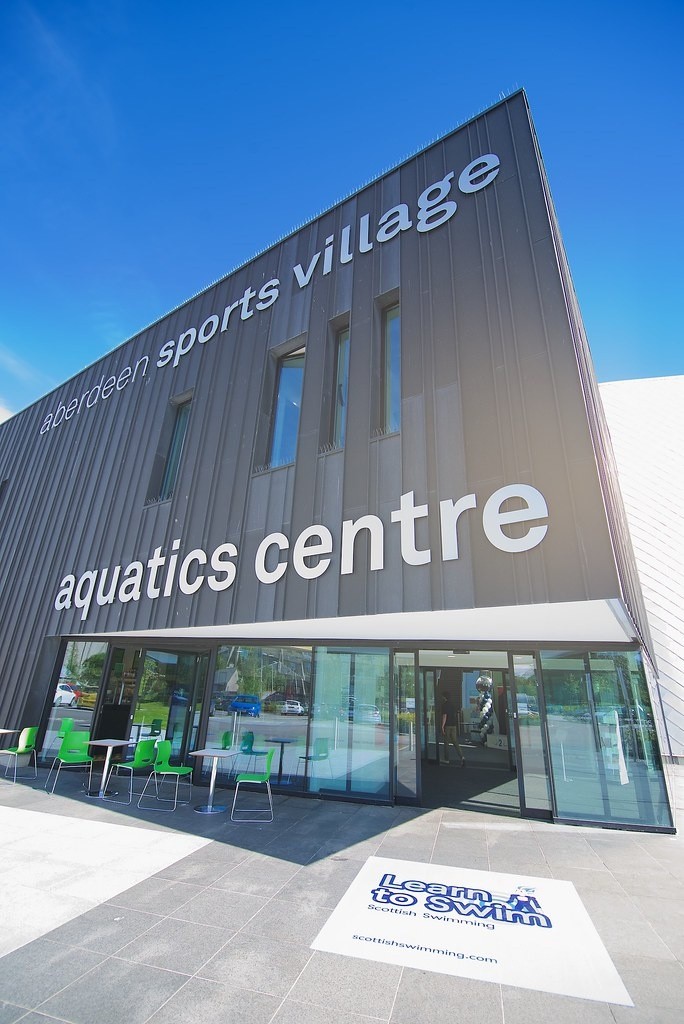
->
[43,731,94,796]
[0,727,39,786]
[102,738,158,805]
[227,731,269,782]
[44,716,75,764]
[141,718,164,741]
[138,740,194,812]
[204,729,234,777]
[230,746,276,823]
[286,737,333,787]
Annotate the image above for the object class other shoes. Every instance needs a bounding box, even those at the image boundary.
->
[440,759,450,764]
[460,757,467,766]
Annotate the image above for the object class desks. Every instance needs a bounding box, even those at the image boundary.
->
[126,723,156,760]
[83,739,137,799]
[188,748,243,814]
[263,736,300,786]
[0,729,21,734]
[77,723,91,728]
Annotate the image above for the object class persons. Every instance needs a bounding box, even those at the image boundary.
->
[441,691,466,767]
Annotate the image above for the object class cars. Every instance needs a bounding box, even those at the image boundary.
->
[53,683,81,707]
[595,705,635,721]
[282,697,381,724]
[227,695,261,718]
[77,686,112,710]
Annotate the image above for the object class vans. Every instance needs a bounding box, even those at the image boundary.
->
[508,693,529,715]
